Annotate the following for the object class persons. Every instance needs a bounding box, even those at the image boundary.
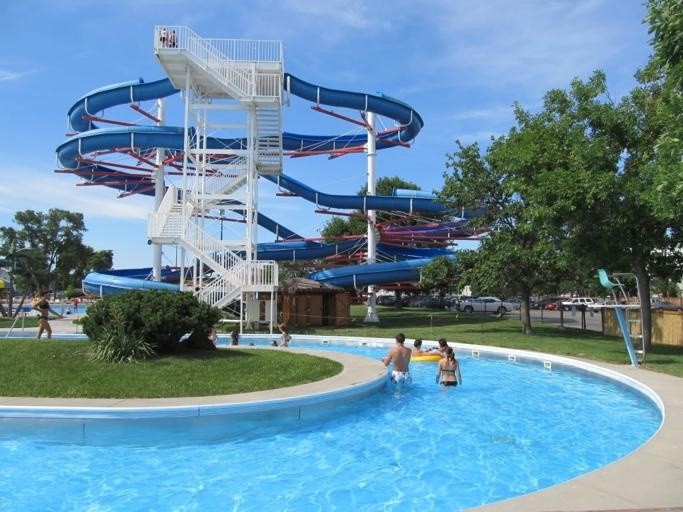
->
[228,329,238,346]
[433,336,449,357]
[208,328,219,345]
[410,338,441,359]
[71,299,78,314]
[29,288,65,341]
[158,28,179,50]
[277,323,292,348]
[270,340,277,346]
[435,346,463,387]
[380,332,414,388]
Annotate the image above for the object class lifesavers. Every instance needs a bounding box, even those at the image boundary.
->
[410,355,441,362]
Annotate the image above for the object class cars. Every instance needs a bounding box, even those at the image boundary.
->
[504,297,683,313]
[376,294,513,314]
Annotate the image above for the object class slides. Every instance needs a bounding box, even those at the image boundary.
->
[54,73,496,316]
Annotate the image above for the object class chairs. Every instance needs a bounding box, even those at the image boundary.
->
[596,268,625,305]
